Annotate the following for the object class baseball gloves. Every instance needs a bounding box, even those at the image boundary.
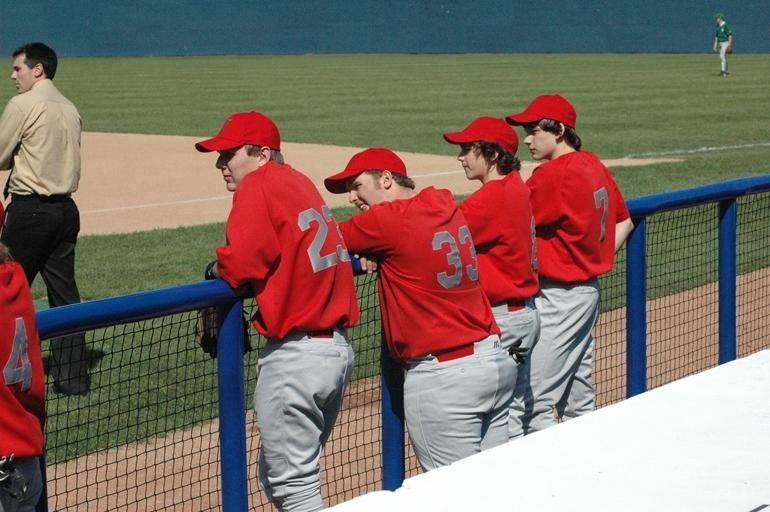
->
[194,259,253,358]
[726,46,732,56]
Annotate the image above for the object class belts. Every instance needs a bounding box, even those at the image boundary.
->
[433,343,474,362]
[309,329,335,339]
[507,300,526,312]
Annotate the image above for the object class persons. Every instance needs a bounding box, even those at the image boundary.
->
[441,116,542,440]
[506,93,637,432]
[709,11,737,75]
[194,111,361,512]
[0,42,94,397]
[325,145,521,471]
[0,204,53,511]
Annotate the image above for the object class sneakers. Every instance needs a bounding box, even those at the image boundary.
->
[720,71,729,76]
[52,373,91,395]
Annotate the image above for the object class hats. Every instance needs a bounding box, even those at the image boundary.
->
[195,111,281,153]
[716,14,724,21]
[444,117,519,157]
[506,94,576,131]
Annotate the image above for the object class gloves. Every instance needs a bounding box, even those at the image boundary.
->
[0,454,15,482]
[507,339,529,365]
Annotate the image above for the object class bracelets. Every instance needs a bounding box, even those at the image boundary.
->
[203,258,218,281]
